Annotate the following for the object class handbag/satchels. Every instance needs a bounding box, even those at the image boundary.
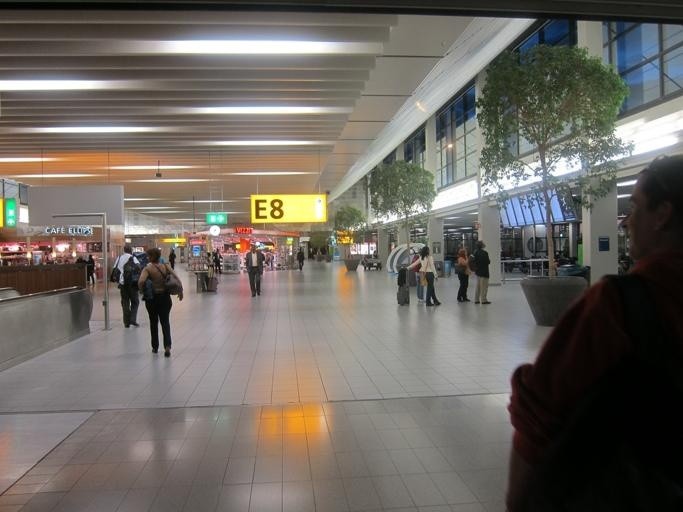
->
[468,254,476,272]
[399,269,406,285]
[454,263,467,274]
[163,274,182,295]
[109,267,120,283]
[123,261,141,289]
[419,278,427,286]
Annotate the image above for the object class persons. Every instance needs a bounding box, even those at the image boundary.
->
[502,151,683,512]
[83,254,95,285]
[473,240,493,304]
[109,245,144,329]
[406,246,441,306]
[212,248,224,274]
[245,244,265,298]
[296,247,305,272]
[137,248,184,357]
[168,250,177,270]
[372,250,378,259]
[454,248,473,303]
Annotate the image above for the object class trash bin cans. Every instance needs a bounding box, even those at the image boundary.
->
[434,261,443,277]
[620,260,628,269]
[558,264,576,276]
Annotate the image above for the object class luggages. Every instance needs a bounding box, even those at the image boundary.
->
[208,264,217,291]
[396,269,409,306]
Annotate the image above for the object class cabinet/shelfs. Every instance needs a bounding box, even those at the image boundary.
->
[187,240,274,275]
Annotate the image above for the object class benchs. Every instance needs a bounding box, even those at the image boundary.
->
[361,257,382,270]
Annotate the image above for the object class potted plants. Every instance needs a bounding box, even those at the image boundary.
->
[367,158,439,286]
[472,45,634,326]
[333,205,366,272]
[309,232,328,262]
